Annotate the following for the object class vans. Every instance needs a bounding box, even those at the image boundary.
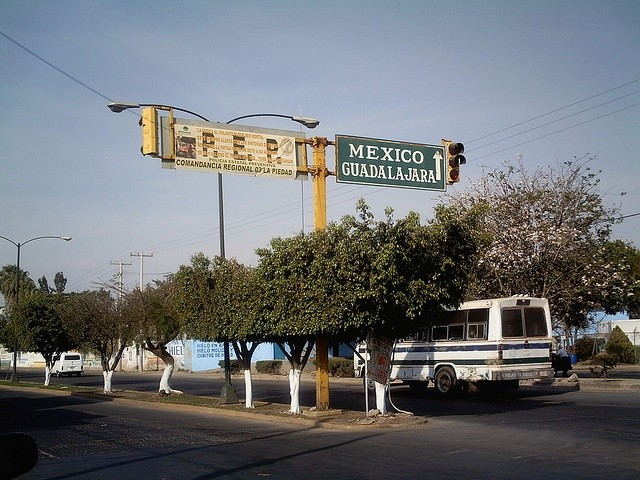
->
[50,351,84,378]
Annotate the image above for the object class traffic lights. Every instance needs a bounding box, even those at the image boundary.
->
[138,108,160,158]
[443,139,466,184]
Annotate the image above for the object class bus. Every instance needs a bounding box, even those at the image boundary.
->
[355,294,554,393]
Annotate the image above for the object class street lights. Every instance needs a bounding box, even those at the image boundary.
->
[107,101,319,405]
[0,236,72,382]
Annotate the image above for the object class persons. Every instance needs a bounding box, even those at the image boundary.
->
[553,343,572,376]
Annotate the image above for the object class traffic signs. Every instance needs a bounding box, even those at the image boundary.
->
[335,134,446,192]
